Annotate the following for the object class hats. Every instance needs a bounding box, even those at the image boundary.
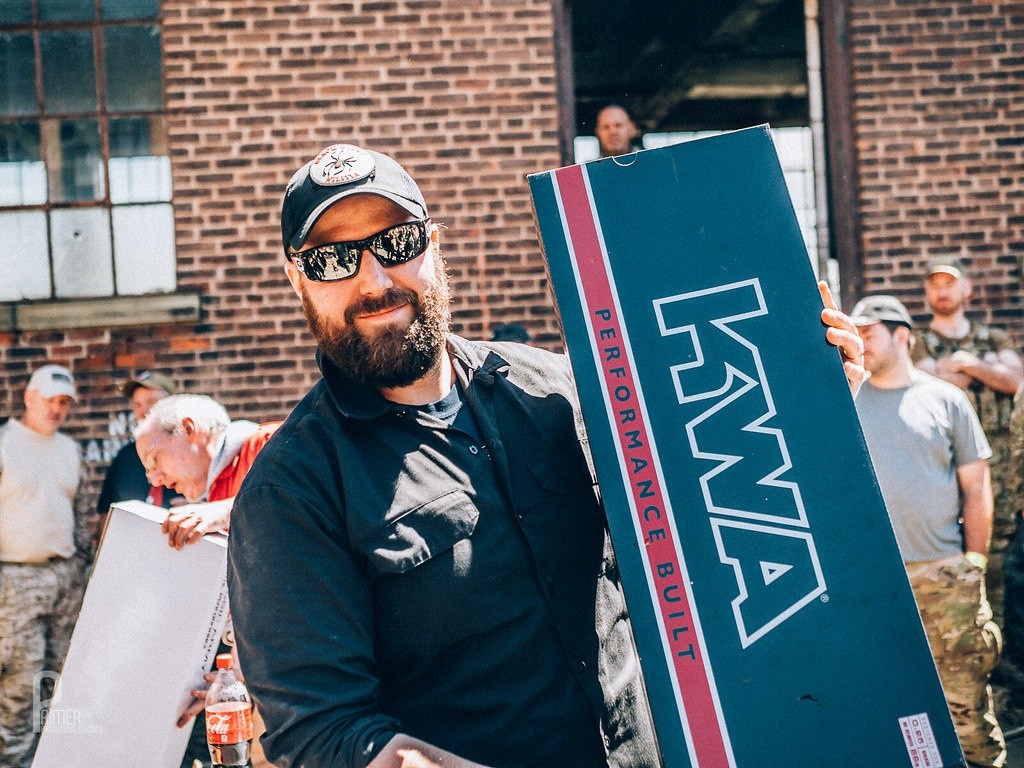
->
[925,254,965,278]
[27,364,79,403]
[849,295,915,327]
[123,371,178,397]
[282,142,431,252]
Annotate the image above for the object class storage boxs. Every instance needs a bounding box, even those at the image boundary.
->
[27,499,230,767]
[527,122,969,767]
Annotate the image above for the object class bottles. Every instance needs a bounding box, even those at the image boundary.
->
[204,654,252,768]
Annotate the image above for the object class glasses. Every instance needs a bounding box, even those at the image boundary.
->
[287,219,431,283]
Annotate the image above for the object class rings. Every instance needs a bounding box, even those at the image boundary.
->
[843,346,865,360]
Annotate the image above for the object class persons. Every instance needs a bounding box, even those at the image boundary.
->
[132,390,289,768]
[847,294,1004,761]
[488,324,534,348]
[94,371,234,768]
[595,102,643,158]
[221,138,870,768]
[904,251,1024,737]
[1,360,105,768]
